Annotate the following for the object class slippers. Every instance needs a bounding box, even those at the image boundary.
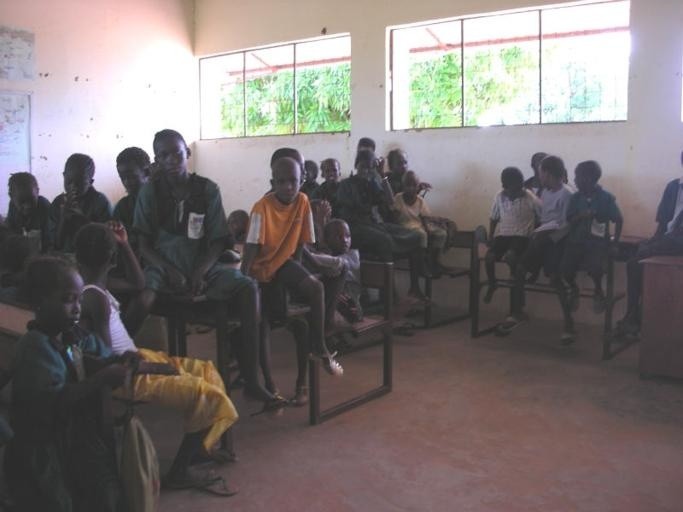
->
[191,470,241,498]
[215,447,240,463]
[249,384,296,418]
[407,288,431,302]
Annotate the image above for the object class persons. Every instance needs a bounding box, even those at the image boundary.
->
[482,151,683,346]
[1,127,290,511]
[229,138,453,406]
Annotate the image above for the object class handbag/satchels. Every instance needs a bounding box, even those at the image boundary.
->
[112,415,163,512]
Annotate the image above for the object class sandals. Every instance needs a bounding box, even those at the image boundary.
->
[319,349,346,377]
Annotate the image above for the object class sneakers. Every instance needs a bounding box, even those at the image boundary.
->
[293,376,311,407]
[496,310,530,334]
[615,312,644,336]
[592,289,606,314]
[558,317,578,345]
[567,286,580,313]
